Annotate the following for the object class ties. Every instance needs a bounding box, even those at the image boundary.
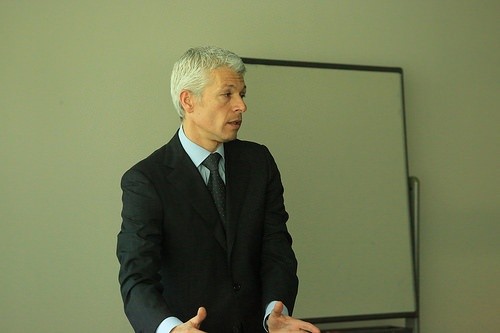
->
[203,153,227,223]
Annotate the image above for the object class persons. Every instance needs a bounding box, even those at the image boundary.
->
[117,46,322,333]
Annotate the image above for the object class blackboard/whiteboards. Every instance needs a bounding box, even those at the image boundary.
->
[214,52,418,321]
